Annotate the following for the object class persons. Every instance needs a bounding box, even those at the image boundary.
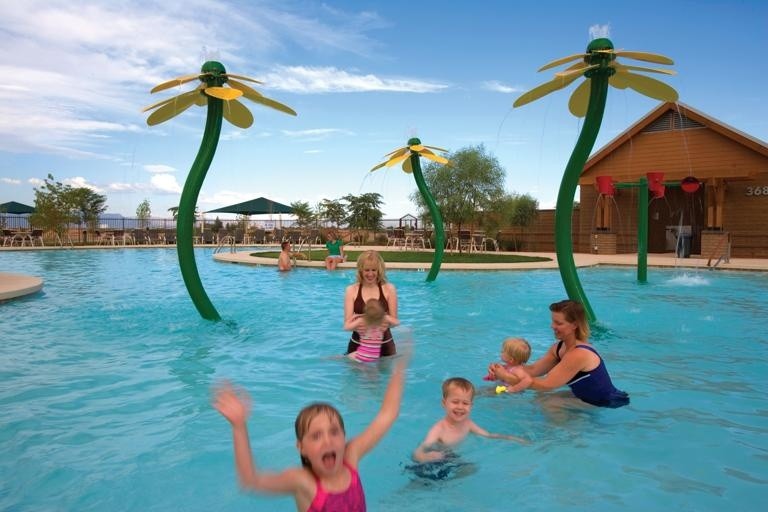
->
[205,339,413,512]
[340,250,400,358]
[474,336,533,400]
[277,242,307,271]
[406,378,537,492]
[324,297,399,364]
[485,298,631,428]
[325,230,346,271]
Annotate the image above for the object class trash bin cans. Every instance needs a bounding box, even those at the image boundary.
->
[675,236,693,258]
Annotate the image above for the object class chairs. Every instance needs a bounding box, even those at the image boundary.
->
[385,226,503,254]
[2,228,45,247]
[95,227,176,246]
[202,227,322,244]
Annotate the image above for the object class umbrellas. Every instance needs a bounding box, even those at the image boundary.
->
[202,196,305,216]
[0,201,39,216]
